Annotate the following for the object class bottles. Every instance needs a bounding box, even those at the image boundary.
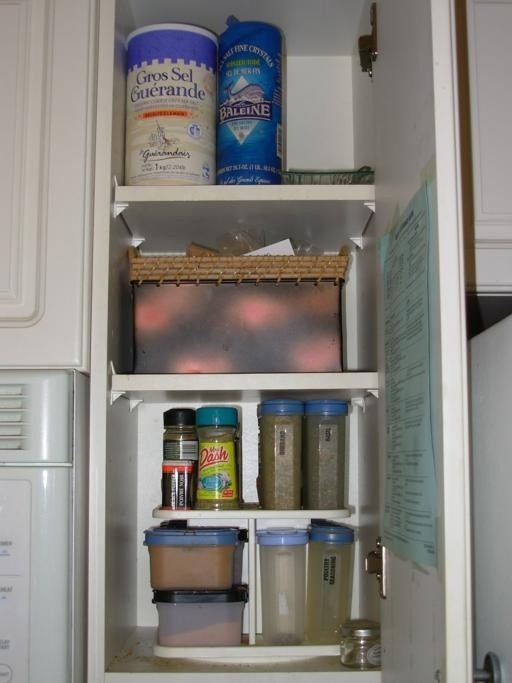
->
[257,520,355,646]
[338,618,382,669]
[159,399,348,508]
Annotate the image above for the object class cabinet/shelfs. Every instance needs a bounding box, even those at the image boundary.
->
[2,1,96,376]
[455,0,512,297]
[86,0,475,681]
